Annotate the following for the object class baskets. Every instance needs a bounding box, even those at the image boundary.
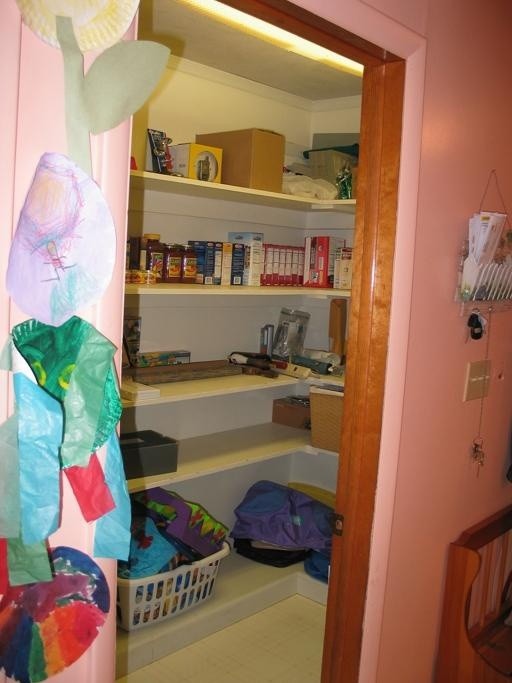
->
[116,539,230,632]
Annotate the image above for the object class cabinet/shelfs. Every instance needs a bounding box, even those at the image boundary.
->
[115,0,363,678]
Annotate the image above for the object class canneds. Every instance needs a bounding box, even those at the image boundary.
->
[126,234,198,284]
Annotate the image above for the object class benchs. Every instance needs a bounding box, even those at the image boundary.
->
[433,503,512,683]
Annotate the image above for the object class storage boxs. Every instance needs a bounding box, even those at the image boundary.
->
[166,142,223,183]
[195,127,285,194]
[120,430,179,479]
